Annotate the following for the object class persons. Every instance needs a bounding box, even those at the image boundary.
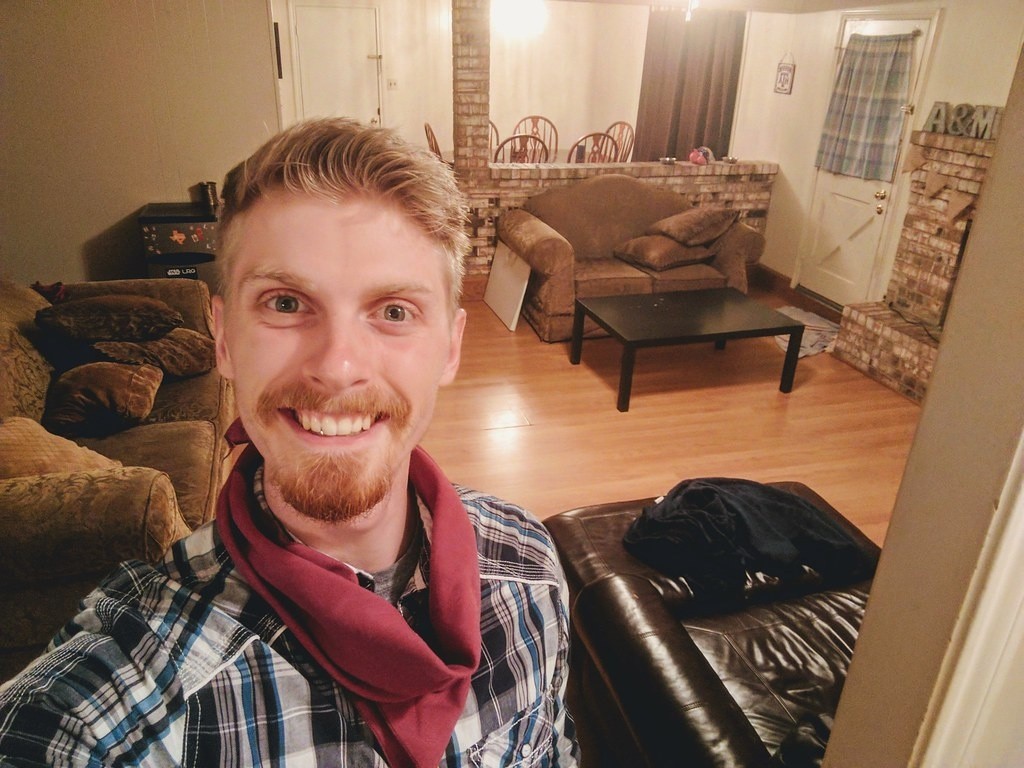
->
[0,115,580,768]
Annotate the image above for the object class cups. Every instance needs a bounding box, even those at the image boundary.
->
[199,181,218,206]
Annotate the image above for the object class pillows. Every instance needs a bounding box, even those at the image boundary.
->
[83,327,219,379]
[612,232,718,272]
[643,205,741,249]
[41,358,164,434]
[34,293,186,344]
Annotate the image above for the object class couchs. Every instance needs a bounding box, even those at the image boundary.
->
[0,278,239,651]
[496,172,766,342]
[539,478,882,768]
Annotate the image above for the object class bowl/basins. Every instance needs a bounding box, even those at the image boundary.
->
[722,156,738,163]
[660,158,676,164]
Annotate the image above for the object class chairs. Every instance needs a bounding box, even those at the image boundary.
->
[423,114,634,171]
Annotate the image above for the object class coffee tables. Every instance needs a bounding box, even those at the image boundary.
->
[568,287,807,414]
